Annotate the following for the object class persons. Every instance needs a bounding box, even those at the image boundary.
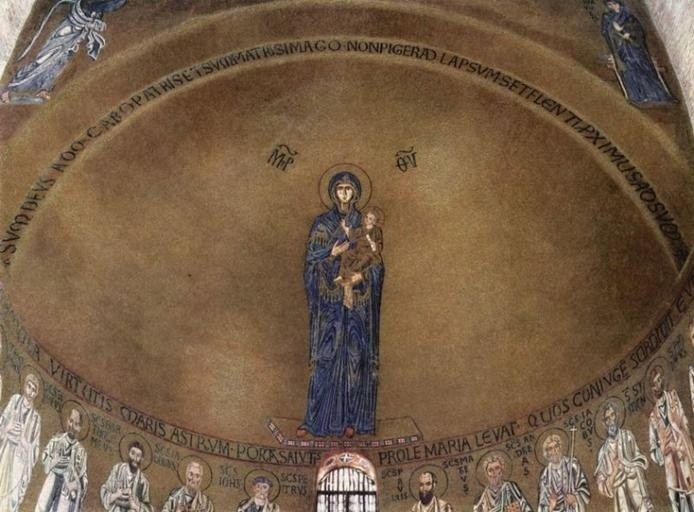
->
[296,163,385,438]
[600,1,680,107]
[1,0,127,105]
[333,205,386,286]
